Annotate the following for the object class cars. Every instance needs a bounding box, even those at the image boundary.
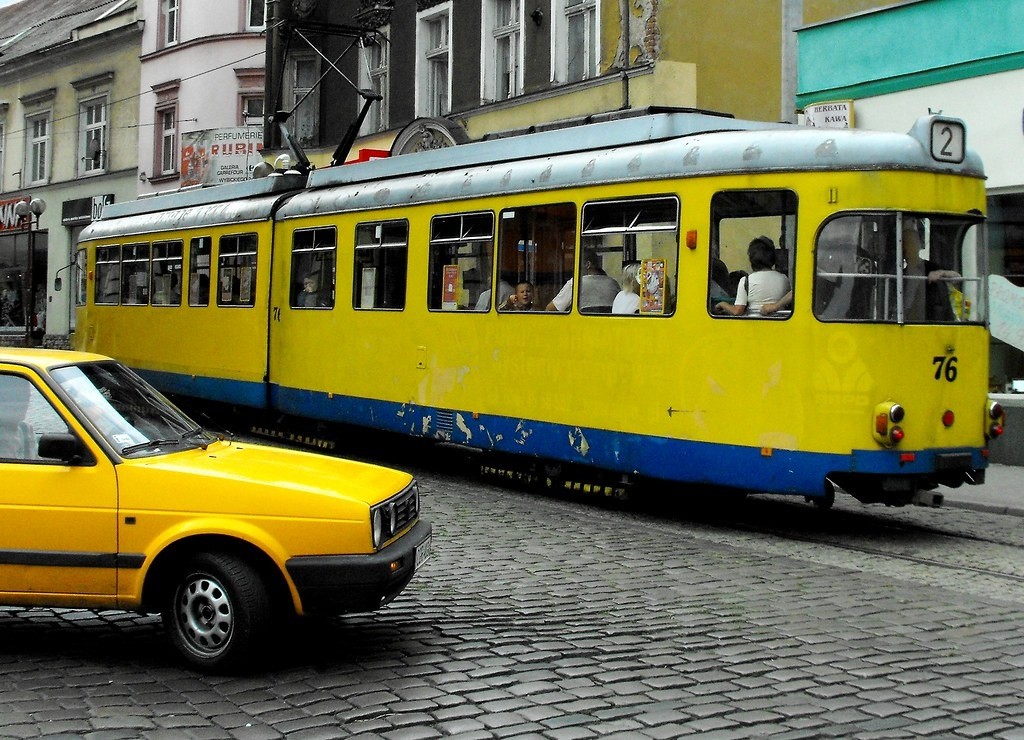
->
[0,347,432,674]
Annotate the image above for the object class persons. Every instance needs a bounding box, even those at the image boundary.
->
[715,228,963,321]
[545,247,621,311]
[498,280,535,311]
[612,262,649,314]
[2,281,18,302]
[152,272,181,305]
[297,273,319,306]
[476,271,517,310]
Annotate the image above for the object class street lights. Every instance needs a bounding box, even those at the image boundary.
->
[15,198,45,336]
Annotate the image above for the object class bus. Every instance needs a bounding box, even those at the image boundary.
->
[54,105,1005,509]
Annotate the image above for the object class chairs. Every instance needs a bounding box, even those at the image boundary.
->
[580,305,613,313]
